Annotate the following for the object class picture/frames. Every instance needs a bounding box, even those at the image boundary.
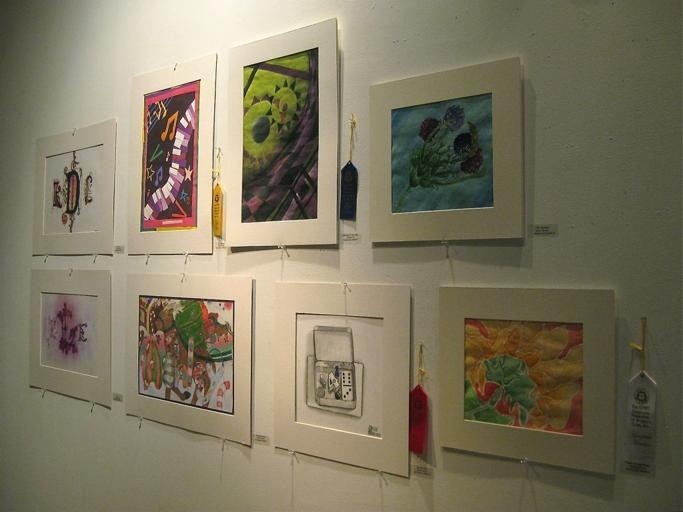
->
[28,269,112,409]
[369,58,524,244]
[437,286,616,475]
[125,273,253,447]
[32,119,116,256]
[224,18,338,247]
[272,280,411,478]
[125,52,217,255]
